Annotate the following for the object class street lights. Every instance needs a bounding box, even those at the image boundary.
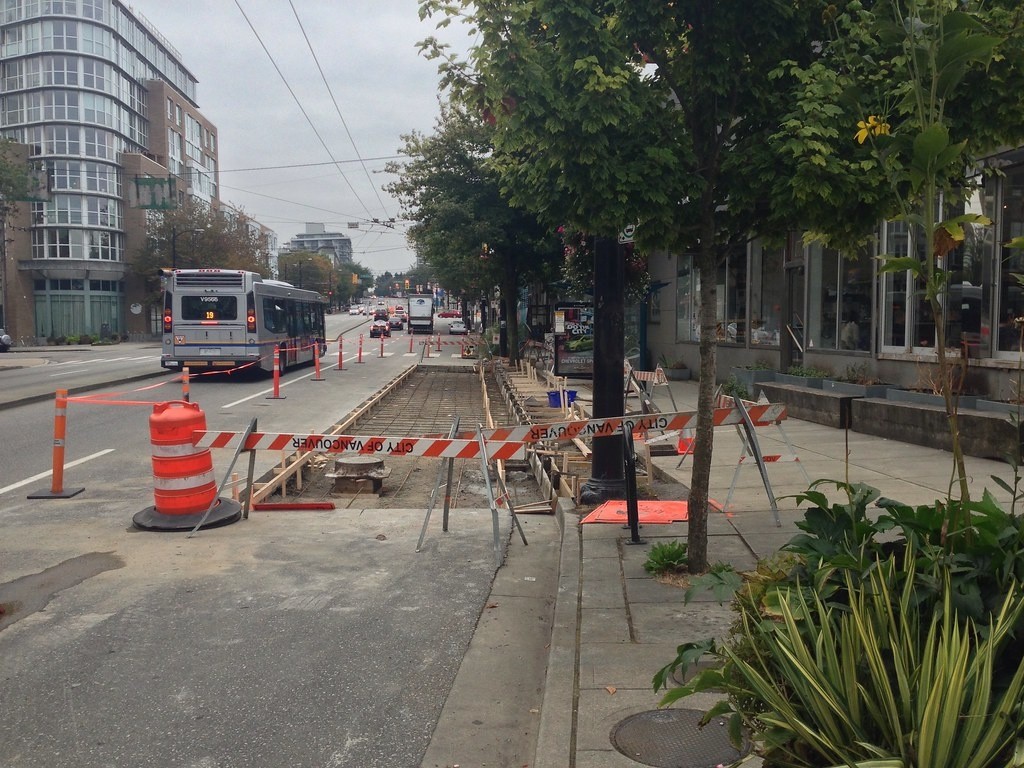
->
[170,226,206,269]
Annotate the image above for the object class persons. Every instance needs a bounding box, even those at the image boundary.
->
[842,310,863,349]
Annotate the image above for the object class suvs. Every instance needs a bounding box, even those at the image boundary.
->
[374,308,390,321]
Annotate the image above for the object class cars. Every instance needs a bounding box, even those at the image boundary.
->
[378,297,384,305]
[396,305,403,310]
[369,320,391,338]
[447,321,467,334]
[438,309,462,319]
[392,310,407,322]
[387,317,403,330]
[344,300,374,316]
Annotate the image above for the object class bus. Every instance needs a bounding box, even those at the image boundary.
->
[157,265,327,380]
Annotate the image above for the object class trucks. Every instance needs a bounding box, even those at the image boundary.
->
[406,293,435,334]
